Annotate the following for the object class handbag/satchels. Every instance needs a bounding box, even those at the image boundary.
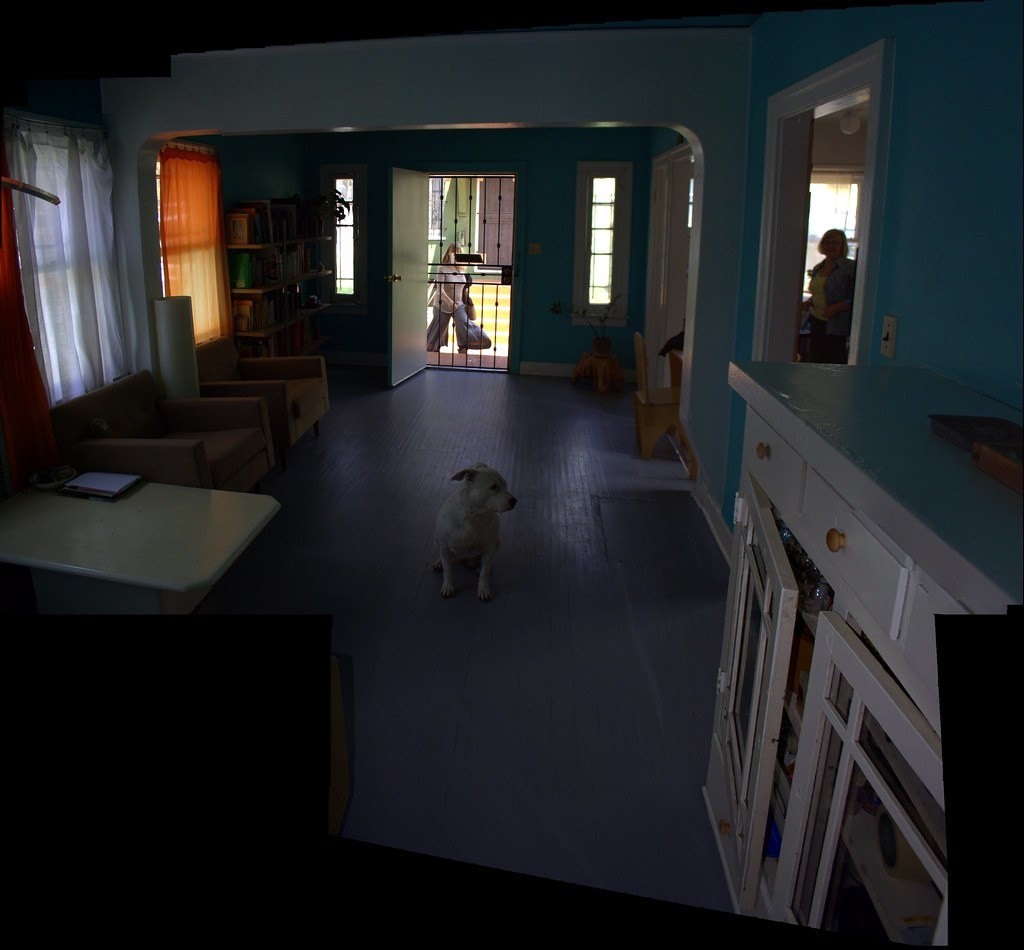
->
[466,320,491,350]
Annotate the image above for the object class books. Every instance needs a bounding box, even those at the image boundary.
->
[225,195,321,357]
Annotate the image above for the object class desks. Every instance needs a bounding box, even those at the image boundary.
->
[668,349,698,482]
[0,480,283,593]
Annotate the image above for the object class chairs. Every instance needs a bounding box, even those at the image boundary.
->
[633,331,686,462]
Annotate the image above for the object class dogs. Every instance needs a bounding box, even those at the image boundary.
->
[432,464,517,603]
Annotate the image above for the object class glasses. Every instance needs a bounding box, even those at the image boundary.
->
[821,240,844,246]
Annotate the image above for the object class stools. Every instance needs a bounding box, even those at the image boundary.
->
[572,351,625,398]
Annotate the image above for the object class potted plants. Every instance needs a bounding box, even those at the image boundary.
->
[547,287,623,359]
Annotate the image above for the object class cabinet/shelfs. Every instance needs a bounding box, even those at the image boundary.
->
[701,360,1024,945]
[226,219,333,357]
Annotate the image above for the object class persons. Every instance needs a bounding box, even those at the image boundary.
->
[426,243,468,354]
[809,230,855,364]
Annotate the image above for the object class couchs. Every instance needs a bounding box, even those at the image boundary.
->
[195,336,329,471]
[48,369,276,492]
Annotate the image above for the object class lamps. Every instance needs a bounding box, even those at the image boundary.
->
[839,107,860,135]
